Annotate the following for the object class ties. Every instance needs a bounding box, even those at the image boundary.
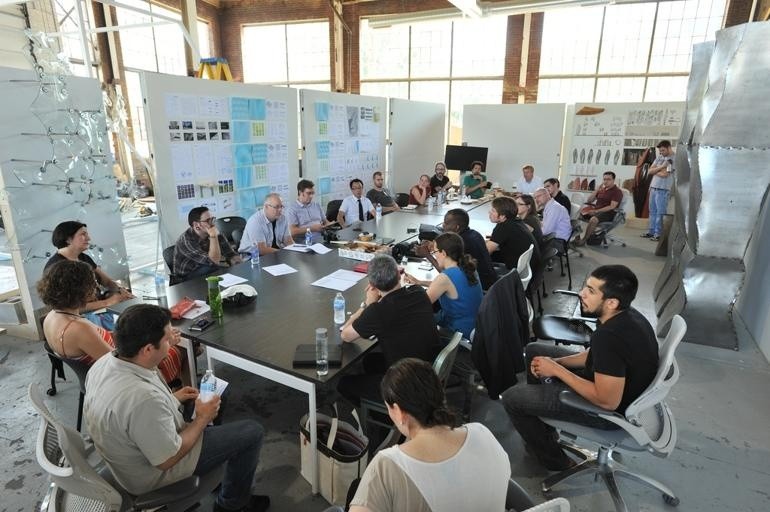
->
[358,200,363,221]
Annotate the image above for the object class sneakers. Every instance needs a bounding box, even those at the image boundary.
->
[640,234,660,241]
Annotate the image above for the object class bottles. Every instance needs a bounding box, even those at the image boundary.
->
[333,292,346,326]
[250,240,260,265]
[154,272,167,299]
[304,227,313,247]
[428,195,434,210]
[315,328,329,377]
[206,277,225,319]
[376,203,382,219]
[438,187,443,206]
[200,370,217,402]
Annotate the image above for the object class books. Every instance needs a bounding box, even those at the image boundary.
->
[294,342,343,367]
[284,242,334,255]
[352,260,406,274]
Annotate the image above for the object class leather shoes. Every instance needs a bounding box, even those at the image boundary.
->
[214,496,270,512]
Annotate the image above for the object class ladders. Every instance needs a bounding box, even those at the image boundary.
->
[198,57,233,81]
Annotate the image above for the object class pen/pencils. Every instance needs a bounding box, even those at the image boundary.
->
[293,245,307,247]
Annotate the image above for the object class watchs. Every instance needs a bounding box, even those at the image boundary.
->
[360,301,369,310]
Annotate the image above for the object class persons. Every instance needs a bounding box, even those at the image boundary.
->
[336,251,442,447]
[344,358,516,512]
[41,219,138,329]
[574,169,627,250]
[404,232,483,353]
[336,178,380,230]
[409,160,574,289]
[640,141,676,242]
[287,180,337,249]
[501,262,663,472]
[77,304,270,512]
[366,172,401,221]
[36,261,204,420]
[171,207,243,284]
[235,193,296,258]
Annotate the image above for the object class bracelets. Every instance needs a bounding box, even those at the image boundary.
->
[117,286,127,294]
[306,225,311,232]
[209,235,219,239]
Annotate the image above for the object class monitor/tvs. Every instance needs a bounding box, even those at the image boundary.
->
[444,145,488,173]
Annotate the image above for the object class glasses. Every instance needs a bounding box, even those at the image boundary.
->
[198,217,216,224]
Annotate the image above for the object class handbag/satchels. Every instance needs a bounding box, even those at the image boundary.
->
[299,398,370,505]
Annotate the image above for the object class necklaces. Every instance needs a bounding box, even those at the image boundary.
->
[53,308,85,320]
